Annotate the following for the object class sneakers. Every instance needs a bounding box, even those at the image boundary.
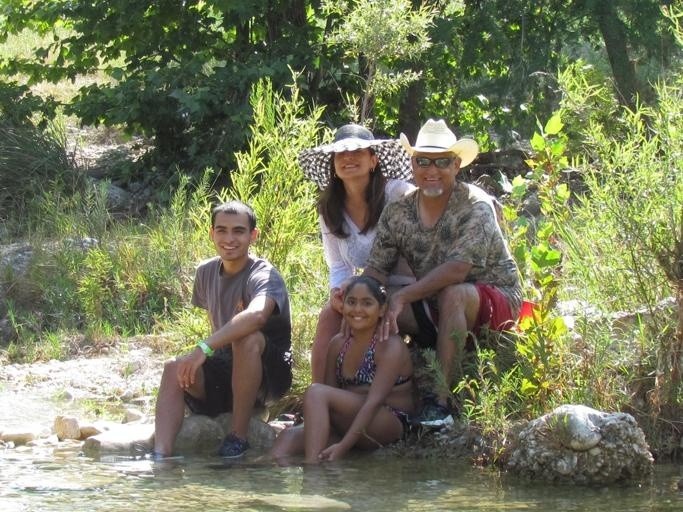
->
[419,404,455,428]
[219,435,249,456]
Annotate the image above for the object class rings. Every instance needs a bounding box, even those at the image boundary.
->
[383,321,391,325]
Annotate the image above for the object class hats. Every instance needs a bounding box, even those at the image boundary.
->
[297,125,414,191]
[400,119,479,168]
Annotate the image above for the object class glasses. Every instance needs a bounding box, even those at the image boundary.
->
[416,157,456,168]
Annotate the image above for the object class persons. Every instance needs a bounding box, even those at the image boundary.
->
[151,200,292,465]
[298,121,422,384]
[252,276,417,466]
[339,118,524,428]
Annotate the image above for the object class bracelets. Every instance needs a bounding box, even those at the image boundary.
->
[195,340,216,358]
